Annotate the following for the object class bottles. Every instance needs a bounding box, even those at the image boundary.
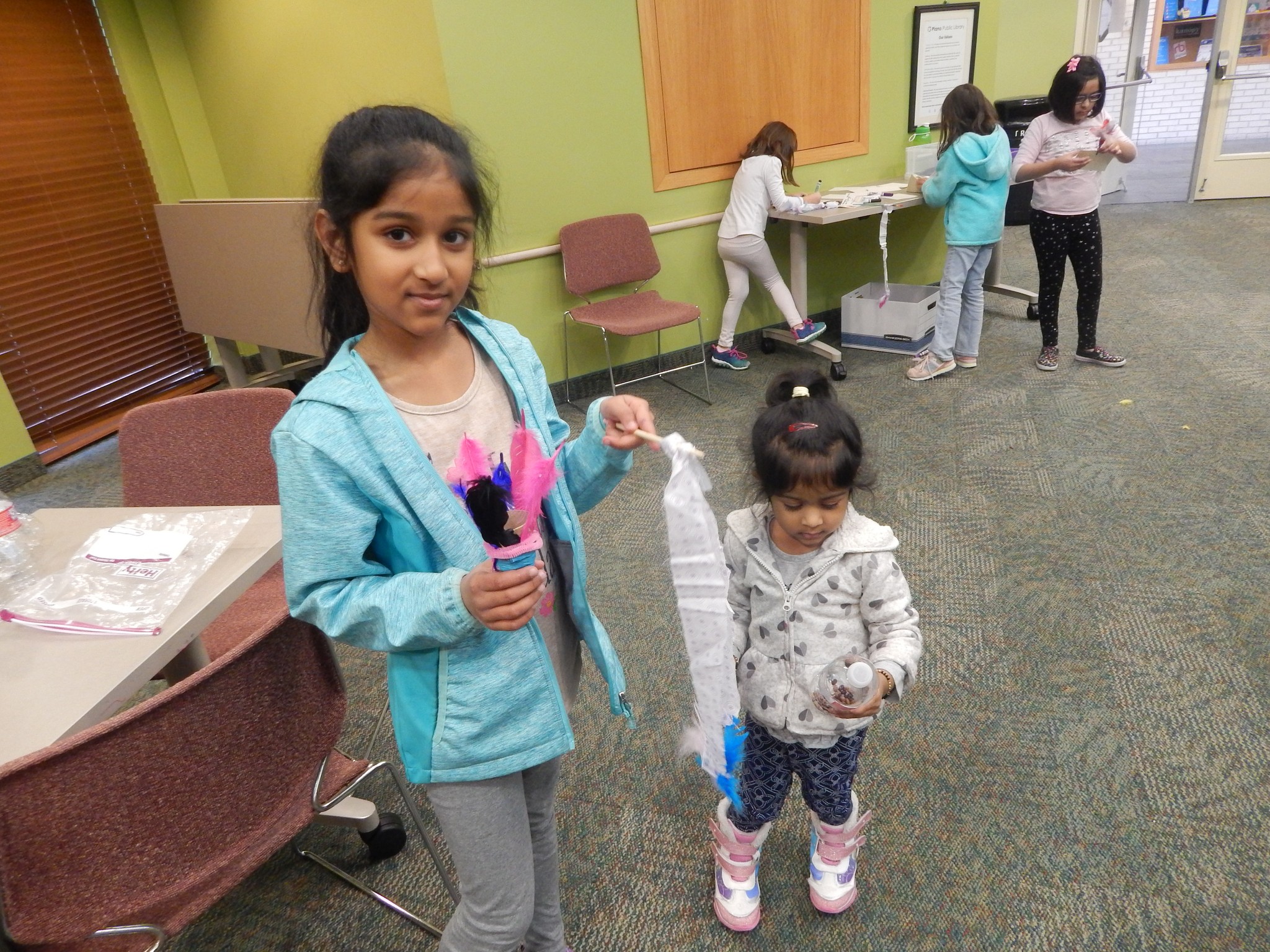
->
[807,653,881,717]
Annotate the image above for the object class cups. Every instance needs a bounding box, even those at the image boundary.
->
[909,123,932,145]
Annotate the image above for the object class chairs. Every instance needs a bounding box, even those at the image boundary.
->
[119,388,296,679]
[557,212,712,414]
[0,600,461,951]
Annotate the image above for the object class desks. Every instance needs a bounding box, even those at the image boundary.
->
[0,507,407,854]
[763,178,1038,380]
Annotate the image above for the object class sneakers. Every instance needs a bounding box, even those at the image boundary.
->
[1036,345,1058,370]
[952,353,977,368]
[708,796,772,931]
[711,344,751,370]
[1075,346,1126,367]
[907,349,957,381]
[790,318,826,346]
[806,790,872,914]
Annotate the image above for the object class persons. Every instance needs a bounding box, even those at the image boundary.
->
[904,84,1012,383]
[1007,54,1137,373]
[710,368,923,932]
[267,104,662,952]
[710,119,827,371]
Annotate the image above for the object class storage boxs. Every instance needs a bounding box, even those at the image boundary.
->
[841,282,941,357]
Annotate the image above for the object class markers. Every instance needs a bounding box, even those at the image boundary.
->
[867,192,893,196]
[863,198,881,203]
[815,180,822,193]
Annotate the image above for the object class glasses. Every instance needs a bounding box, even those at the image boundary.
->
[1076,92,1102,104]
